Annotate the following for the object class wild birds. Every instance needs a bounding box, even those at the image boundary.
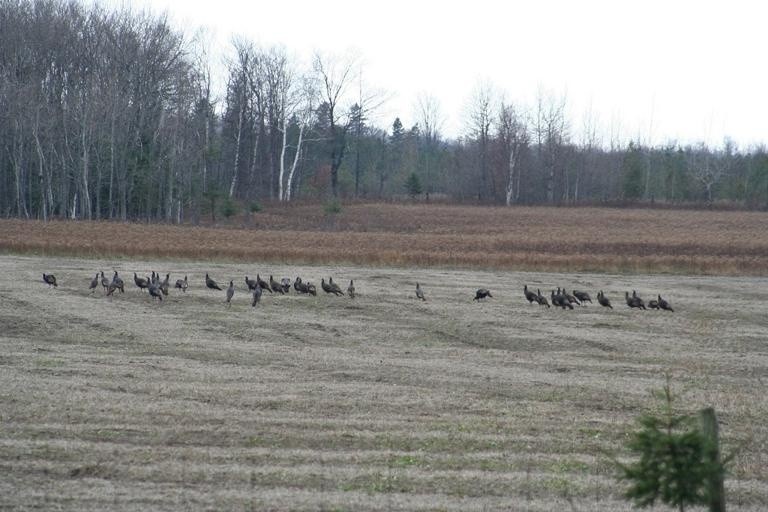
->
[226,280,234,303]
[244,272,356,299]
[473,287,492,303]
[205,271,222,291]
[414,281,427,302]
[42,272,58,289]
[251,284,262,307]
[523,284,613,311]
[625,289,675,313]
[88,269,189,302]
